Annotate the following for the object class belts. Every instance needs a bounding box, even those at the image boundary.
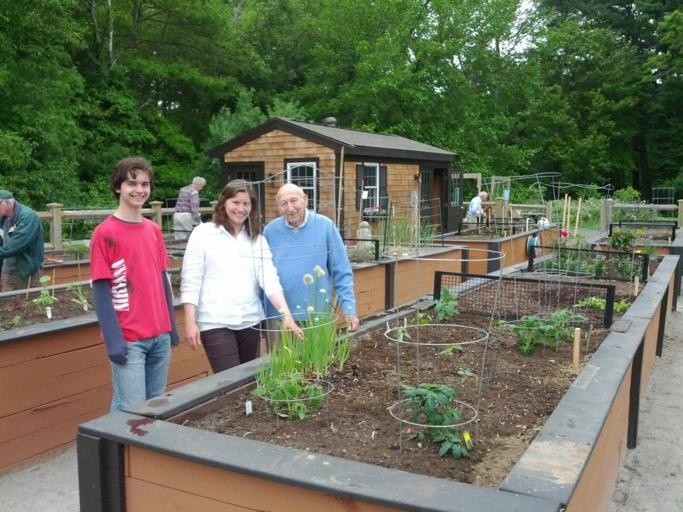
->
[174,210,190,213]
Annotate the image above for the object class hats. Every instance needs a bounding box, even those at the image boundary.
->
[0,189,13,200]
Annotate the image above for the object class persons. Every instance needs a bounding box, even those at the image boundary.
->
[86,156,180,414]
[465,190,489,220]
[257,181,361,356]
[0,189,43,295]
[177,177,304,378]
[171,176,209,241]
